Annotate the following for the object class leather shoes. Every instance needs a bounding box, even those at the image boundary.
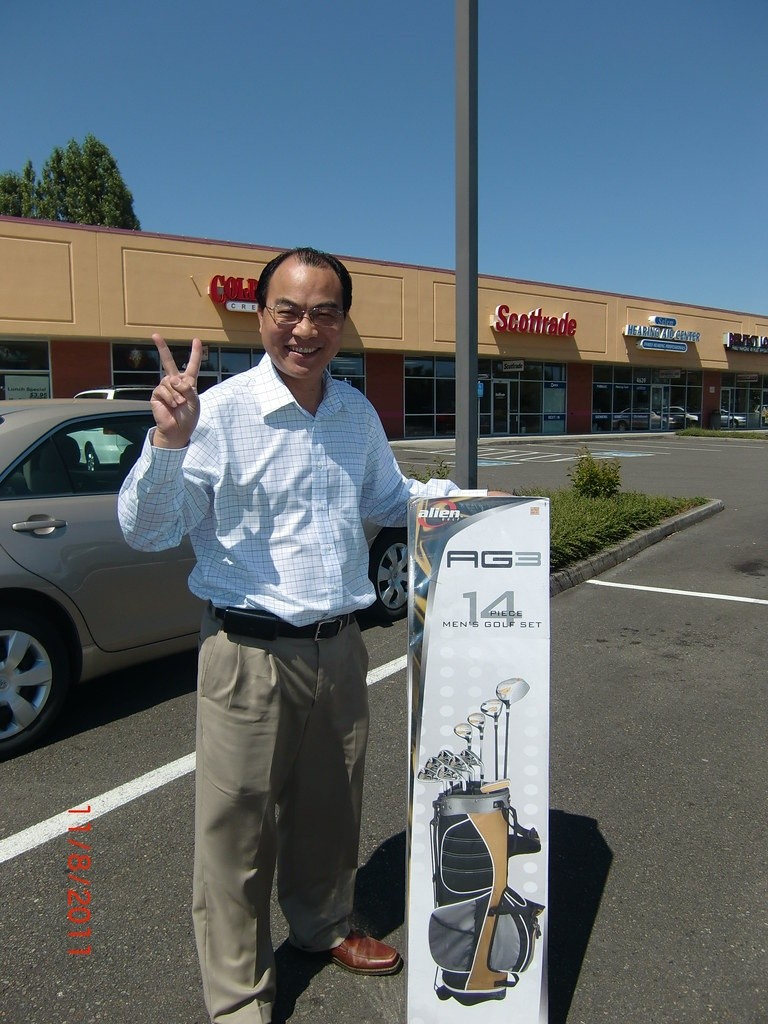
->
[313,928,401,975]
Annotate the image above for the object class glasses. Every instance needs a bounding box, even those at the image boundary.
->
[264,303,347,328]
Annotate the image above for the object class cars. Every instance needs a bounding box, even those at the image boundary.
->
[657,406,700,429]
[0,392,416,765]
[64,385,162,472]
[754,405,768,425]
[609,408,676,432]
[719,409,747,430]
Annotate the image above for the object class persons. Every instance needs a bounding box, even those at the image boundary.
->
[118,249,510,1023]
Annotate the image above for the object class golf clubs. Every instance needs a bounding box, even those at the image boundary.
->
[416,675,531,796]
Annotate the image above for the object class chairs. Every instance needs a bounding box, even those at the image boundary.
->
[111,444,141,491]
[30,436,96,495]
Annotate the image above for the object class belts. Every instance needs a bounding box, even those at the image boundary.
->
[207,604,357,641]
